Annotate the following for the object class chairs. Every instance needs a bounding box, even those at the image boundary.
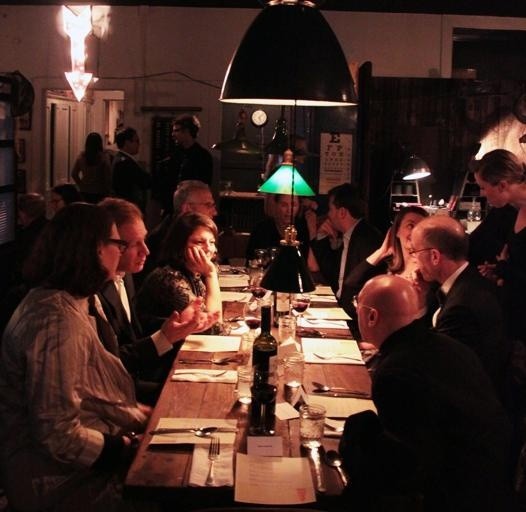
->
[217,231,255,270]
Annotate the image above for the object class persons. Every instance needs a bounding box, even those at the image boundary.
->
[312,150,525,510]
[2,110,224,511]
[247,193,319,276]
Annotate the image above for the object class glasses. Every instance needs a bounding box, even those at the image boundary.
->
[172,129,183,132]
[189,202,216,208]
[408,247,433,257]
[103,238,129,253]
[352,296,373,313]
[49,199,62,203]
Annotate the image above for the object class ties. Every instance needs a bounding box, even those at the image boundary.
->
[117,279,131,322]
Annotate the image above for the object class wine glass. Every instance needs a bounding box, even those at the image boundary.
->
[290,293,310,329]
[244,303,262,342]
[250,273,267,313]
[254,247,268,279]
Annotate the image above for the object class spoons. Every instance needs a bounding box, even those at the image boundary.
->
[324,449,348,486]
[148,425,217,438]
[303,316,345,328]
[178,356,232,366]
[302,439,327,492]
[310,381,367,396]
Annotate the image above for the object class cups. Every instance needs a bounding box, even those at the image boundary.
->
[284,352,304,387]
[277,314,296,343]
[299,406,327,442]
[236,366,254,405]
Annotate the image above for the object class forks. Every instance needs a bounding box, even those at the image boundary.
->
[204,437,220,486]
[314,352,361,363]
[174,371,229,378]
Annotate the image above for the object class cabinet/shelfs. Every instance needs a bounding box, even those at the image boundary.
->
[351,60,526,205]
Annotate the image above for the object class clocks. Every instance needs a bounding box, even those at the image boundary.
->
[250,109,268,128]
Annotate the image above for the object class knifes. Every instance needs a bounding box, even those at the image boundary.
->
[215,426,240,434]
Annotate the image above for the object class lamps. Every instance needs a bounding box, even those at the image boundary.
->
[257,105,315,198]
[256,105,308,158]
[401,152,431,206]
[216,0,360,106]
[207,105,263,155]
[254,99,315,294]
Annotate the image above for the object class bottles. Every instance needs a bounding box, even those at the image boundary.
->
[248,306,279,434]
[467,196,483,235]
